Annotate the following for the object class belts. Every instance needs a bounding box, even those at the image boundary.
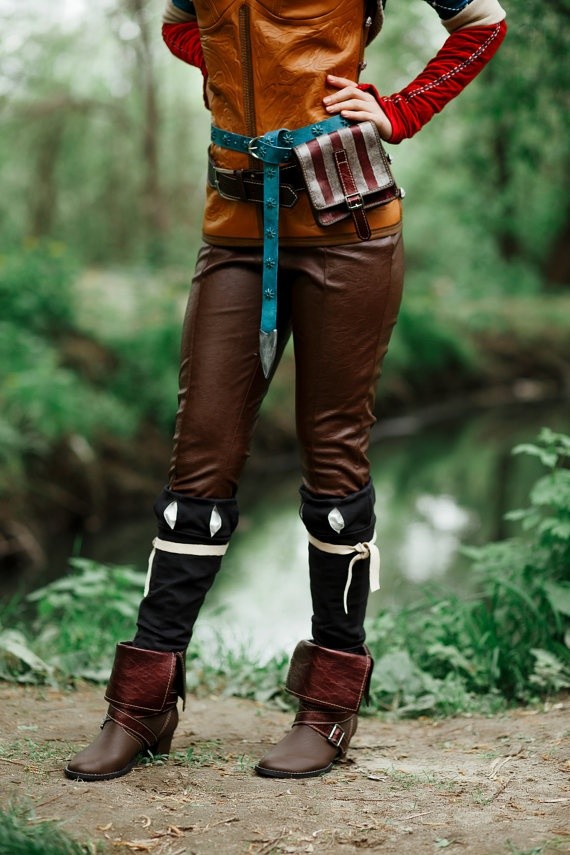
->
[207,164,306,209]
[210,114,353,380]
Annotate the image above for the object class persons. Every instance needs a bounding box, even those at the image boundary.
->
[67,0,505,780]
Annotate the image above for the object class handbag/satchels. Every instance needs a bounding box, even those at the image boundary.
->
[294,121,400,243]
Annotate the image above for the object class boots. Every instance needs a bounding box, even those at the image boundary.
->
[63,642,186,782]
[254,641,374,778]
